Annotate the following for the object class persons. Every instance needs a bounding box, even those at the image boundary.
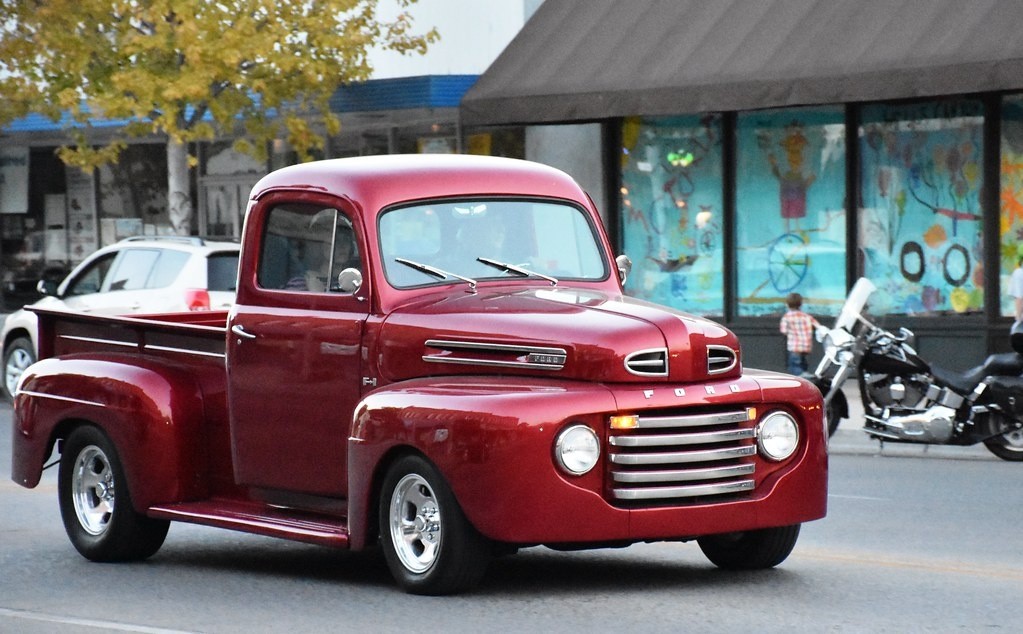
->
[304,216,356,292]
[780,292,821,376]
[1007,259,1023,356]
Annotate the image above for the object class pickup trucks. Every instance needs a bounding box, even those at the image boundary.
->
[10,154,830,599]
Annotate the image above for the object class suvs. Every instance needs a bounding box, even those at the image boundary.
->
[0,234,242,407]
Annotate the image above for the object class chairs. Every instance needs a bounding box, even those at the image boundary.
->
[261,236,326,290]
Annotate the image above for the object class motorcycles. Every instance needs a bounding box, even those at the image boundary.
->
[796,276,1023,462]
